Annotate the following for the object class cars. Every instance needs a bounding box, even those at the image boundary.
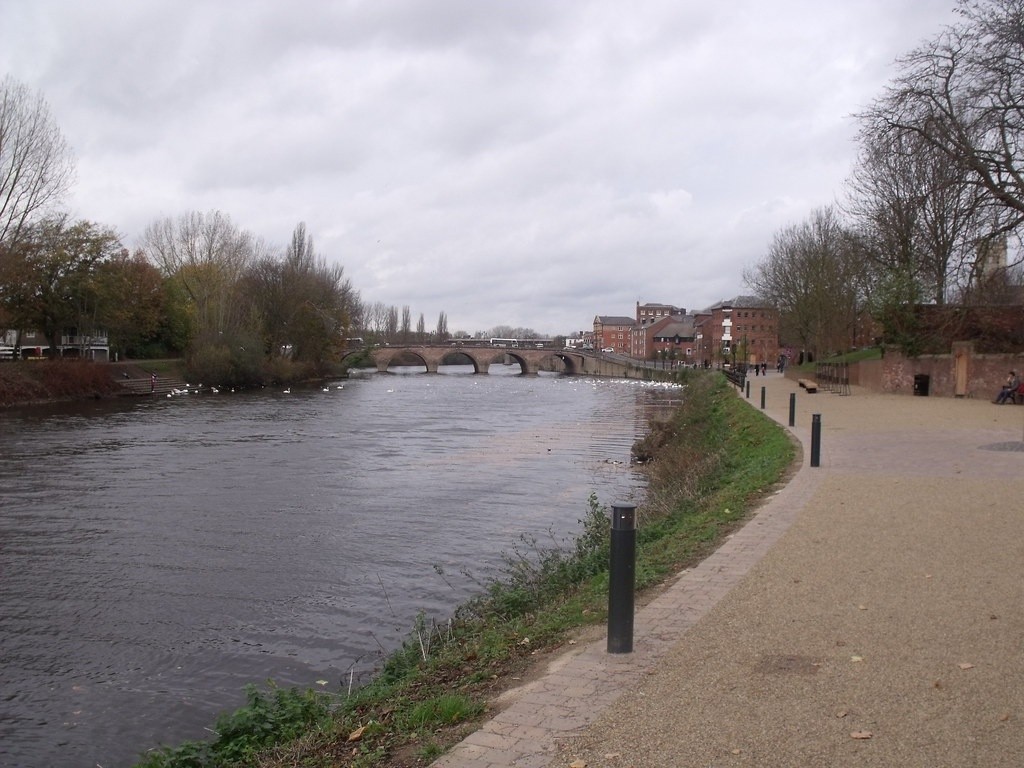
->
[602,347,614,353]
[537,344,543,348]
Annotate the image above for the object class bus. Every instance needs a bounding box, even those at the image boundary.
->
[490,338,518,348]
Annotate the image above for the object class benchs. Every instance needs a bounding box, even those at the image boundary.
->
[797,379,817,394]
[1002,386,1024,404]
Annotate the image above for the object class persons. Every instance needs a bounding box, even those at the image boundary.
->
[992,371,1024,404]
[704,358,708,369]
[755,363,759,376]
[778,361,784,372]
[151,372,156,392]
[762,360,767,375]
[694,362,696,369]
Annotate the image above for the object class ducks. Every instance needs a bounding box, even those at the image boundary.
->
[337,385,344,389]
[323,387,330,392]
[283,387,291,393]
[167,385,235,398]
[386,389,394,393]
[555,375,685,390]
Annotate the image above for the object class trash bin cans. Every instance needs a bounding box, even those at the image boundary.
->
[678,361,685,367]
[913,374,929,397]
